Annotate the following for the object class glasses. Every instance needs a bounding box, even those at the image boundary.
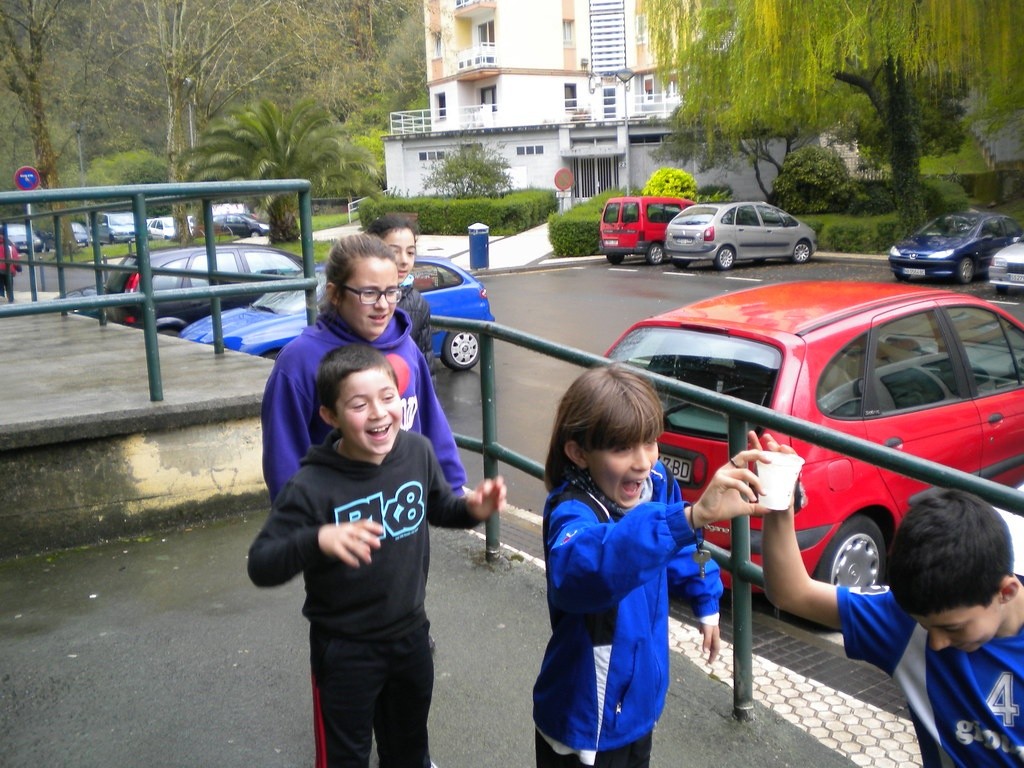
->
[329,280,402,304]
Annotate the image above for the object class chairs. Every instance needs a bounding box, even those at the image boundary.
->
[676,331,953,421]
[414,276,432,290]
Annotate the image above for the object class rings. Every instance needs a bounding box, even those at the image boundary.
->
[730,458,739,468]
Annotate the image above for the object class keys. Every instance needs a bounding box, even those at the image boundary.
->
[693,548,711,578]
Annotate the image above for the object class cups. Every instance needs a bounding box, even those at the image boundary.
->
[752,451,805,511]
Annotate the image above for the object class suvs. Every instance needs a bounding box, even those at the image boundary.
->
[664,200,818,272]
[106,243,327,337]
[598,196,697,266]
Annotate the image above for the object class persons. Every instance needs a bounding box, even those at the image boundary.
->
[531,363,772,768]
[261,232,467,515]
[367,216,437,394]
[246,343,507,768]
[0,226,23,305]
[747,430,1024,768]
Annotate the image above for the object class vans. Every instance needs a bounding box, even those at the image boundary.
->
[96,211,137,246]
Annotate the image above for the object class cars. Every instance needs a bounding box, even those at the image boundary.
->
[602,280,1023,634]
[147,215,195,240]
[177,253,496,373]
[988,233,1024,295]
[888,211,1024,286]
[214,212,270,239]
[53,282,106,319]
[70,222,90,247]
[0,223,44,254]
